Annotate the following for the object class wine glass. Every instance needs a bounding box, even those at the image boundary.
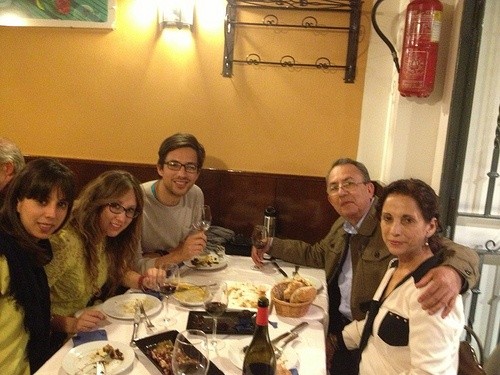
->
[157,263,227,350]
[192,205,211,256]
[252,225,270,271]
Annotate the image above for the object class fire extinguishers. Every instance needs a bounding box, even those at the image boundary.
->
[399,0,444,100]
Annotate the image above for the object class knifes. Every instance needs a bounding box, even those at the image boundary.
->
[271,321,308,344]
[271,261,288,278]
[130,307,140,346]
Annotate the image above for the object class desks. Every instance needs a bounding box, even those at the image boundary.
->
[33,255,329,375]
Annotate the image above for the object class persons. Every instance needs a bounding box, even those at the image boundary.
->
[0,137,26,192]
[327,177,465,375]
[0,156,105,375]
[249,159,480,375]
[42,170,167,315]
[130,133,206,270]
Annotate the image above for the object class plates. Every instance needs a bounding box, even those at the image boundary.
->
[62,340,135,375]
[228,338,297,371]
[102,293,162,320]
[136,330,225,375]
[171,252,273,312]
[274,272,323,290]
[185,311,258,334]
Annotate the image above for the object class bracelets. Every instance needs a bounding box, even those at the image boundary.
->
[50,313,58,320]
[72,318,79,332]
[330,332,337,351]
[138,276,144,288]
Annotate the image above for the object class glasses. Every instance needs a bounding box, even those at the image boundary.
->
[105,202,139,219]
[162,161,200,174]
[326,181,364,197]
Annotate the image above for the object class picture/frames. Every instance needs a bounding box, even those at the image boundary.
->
[0,0,116,29]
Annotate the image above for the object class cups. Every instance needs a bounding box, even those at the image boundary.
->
[172,329,210,375]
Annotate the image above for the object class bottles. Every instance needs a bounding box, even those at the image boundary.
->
[263,206,277,236]
[242,296,276,375]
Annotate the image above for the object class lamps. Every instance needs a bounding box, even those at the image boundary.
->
[158,0,194,30]
